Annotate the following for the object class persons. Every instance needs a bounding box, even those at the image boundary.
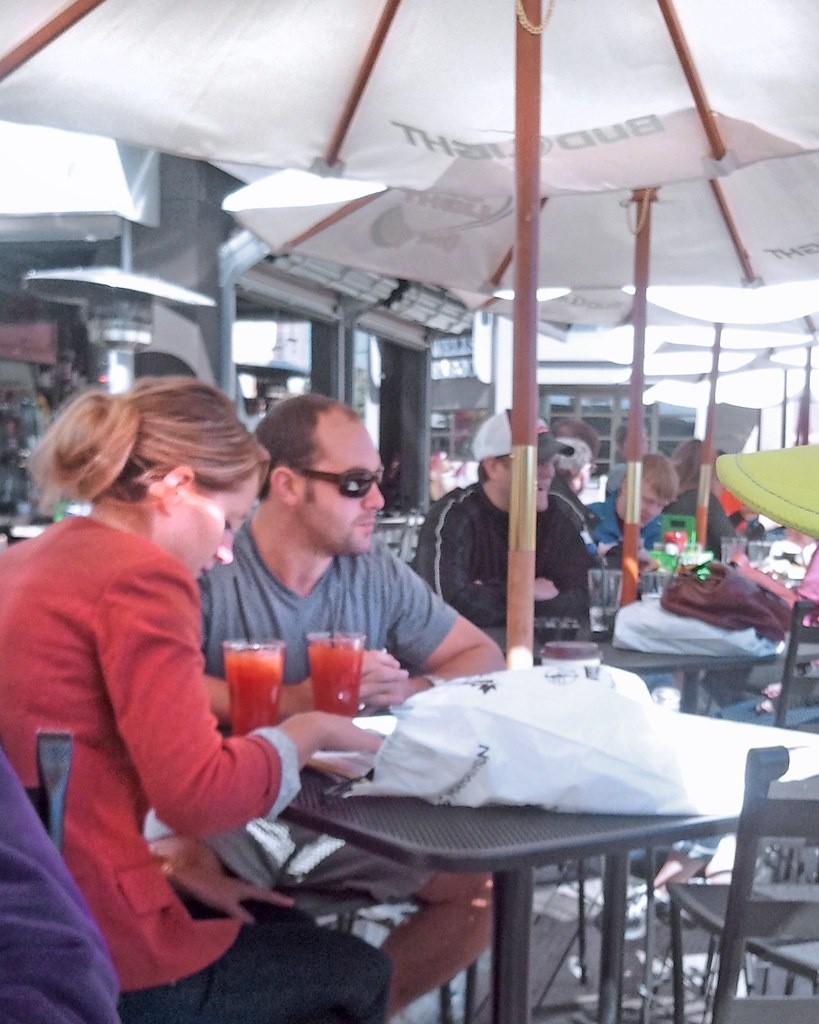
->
[620,444,819,942]
[411,407,615,641]
[554,431,604,529]
[708,449,765,541]
[663,438,752,569]
[606,425,650,495]
[585,453,679,573]
[0,364,398,1024]
[144,390,506,1024]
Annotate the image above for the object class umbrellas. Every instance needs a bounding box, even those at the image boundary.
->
[1,0,818,675]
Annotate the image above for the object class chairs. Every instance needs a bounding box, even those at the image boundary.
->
[664,746,819,1024]
[282,886,453,1024]
[718,600,819,735]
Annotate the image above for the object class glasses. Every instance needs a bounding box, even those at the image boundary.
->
[293,463,384,499]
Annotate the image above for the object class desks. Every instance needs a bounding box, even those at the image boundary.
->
[486,630,819,713]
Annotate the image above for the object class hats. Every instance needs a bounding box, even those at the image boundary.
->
[472,410,574,466]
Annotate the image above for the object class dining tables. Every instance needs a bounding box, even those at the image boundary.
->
[281,766,819,1024]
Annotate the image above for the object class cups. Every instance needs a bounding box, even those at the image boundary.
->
[586,568,624,633]
[222,638,287,738]
[639,570,673,600]
[539,640,603,676]
[303,631,366,717]
[721,536,747,565]
[747,540,771,564]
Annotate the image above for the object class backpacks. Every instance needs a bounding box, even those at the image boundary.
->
[661,560,794,642]
